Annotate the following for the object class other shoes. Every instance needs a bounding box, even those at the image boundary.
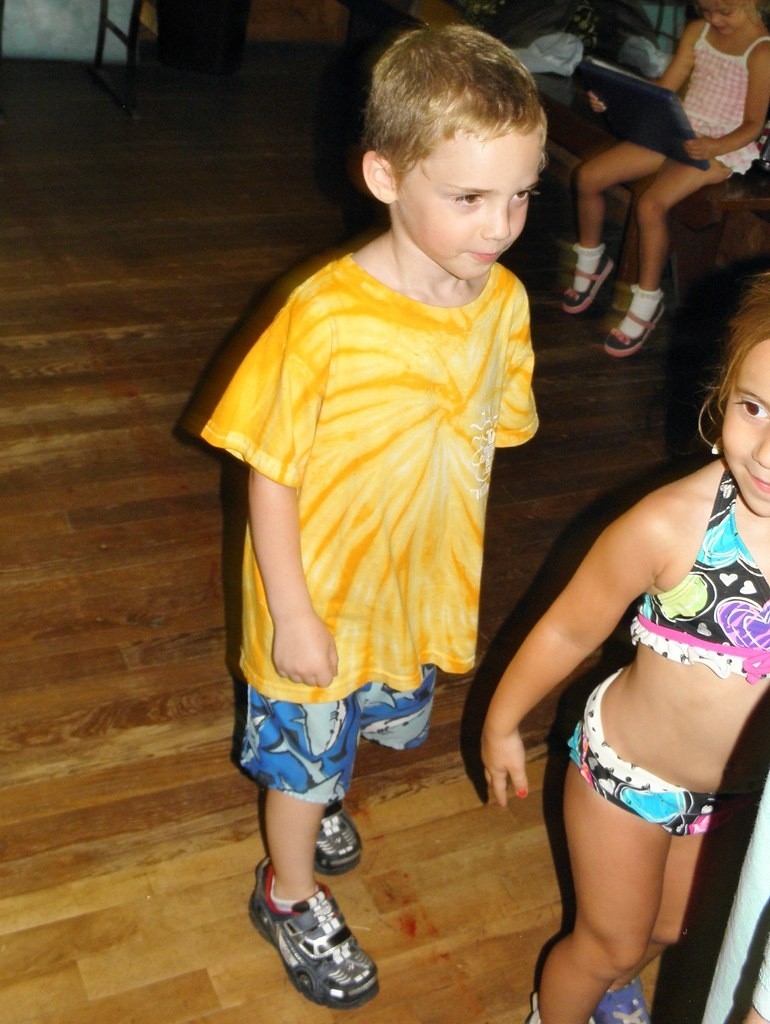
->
[562,252,615,312]
[603,290,665,357]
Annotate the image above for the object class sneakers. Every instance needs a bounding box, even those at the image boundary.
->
[312,795,364,875]
[248,856,379,1010]
[529,976,653,1024]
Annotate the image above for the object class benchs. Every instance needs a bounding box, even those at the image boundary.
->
[520,70,770,335]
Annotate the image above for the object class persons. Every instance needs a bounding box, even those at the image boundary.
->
[199,24,542,1011]
[560,0,770,357]
[481,266,770,1024]
[391,1,599,82]
